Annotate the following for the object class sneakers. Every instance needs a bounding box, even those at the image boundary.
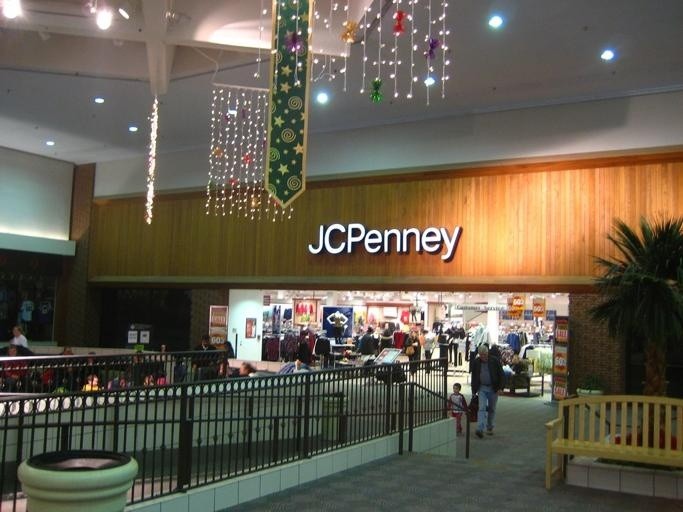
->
[454,428,492,440]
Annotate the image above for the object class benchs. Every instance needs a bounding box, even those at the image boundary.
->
[545,393,683,490]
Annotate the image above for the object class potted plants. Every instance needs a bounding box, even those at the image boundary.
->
[564,214,683,501]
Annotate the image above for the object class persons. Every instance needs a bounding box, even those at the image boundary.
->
[0,344,228,395]
[429,318,478,369]
[510,354,527,375]
[223,341,235,359]
[325,310,349,344]
[420,329,437,374]
[359,325,377,377]
[405,331,421,376]
[191,333,216,367]
[290,354,308,384]
[236,362,256,377]
[469,344,506,439]
[446,383,467,436]
[8,325,28,349]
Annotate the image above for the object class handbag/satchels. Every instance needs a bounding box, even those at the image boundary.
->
[467,395,479,422]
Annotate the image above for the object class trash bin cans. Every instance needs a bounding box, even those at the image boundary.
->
[16,450,139,512]
[128,324,152,350]
[323,396,349,441]
